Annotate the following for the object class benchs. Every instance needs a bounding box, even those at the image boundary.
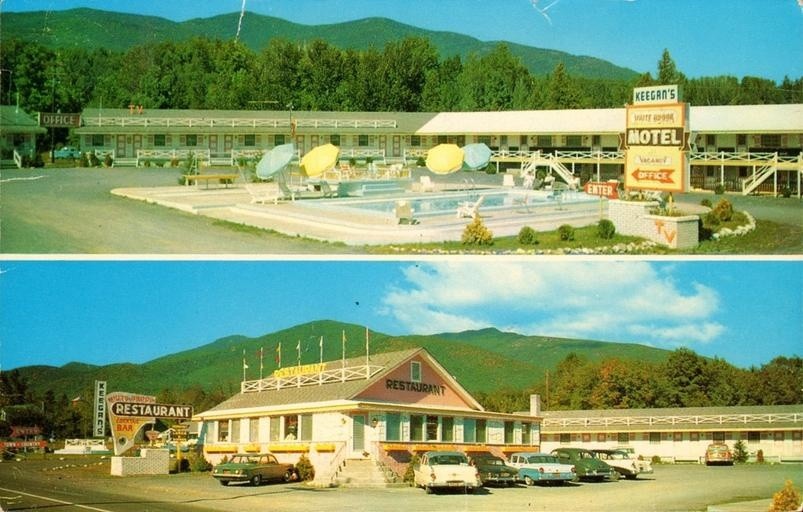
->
[182,173,240,189]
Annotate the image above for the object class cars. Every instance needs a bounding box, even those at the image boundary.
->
[211,454,294,486]
[705,443,732,465]
[412,447,653,494]
[50,146,79,158]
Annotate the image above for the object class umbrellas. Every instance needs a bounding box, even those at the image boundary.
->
[461,143,493,170]
[424,143,465,175]
[299,142,341,179]
[255,143,294,180]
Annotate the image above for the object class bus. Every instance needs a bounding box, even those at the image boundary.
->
[161,432,198,454]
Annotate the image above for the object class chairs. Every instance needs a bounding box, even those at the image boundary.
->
[418,175,440,192]
[319,181,337,198]
[333,159,403,184]
[500,173,581,214]
[391,199,419,226]
[244,181,303,206]
[456,197,485,221]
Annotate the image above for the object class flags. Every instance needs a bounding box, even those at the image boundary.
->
[257,350,263,356]
[274,344,282,363]
